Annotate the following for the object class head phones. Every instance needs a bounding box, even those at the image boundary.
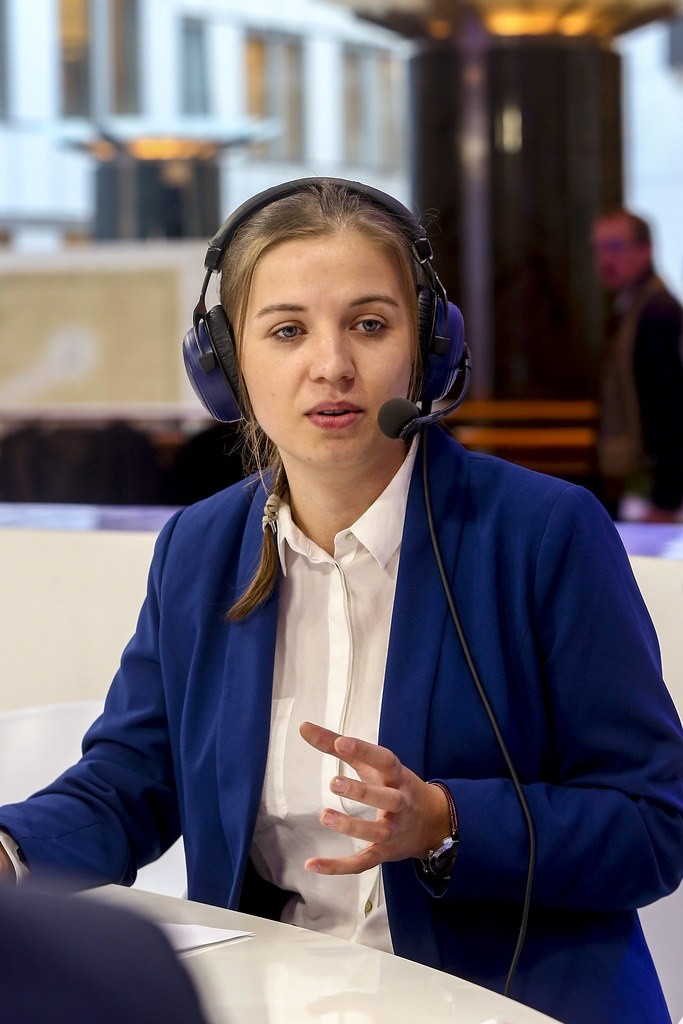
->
[182,176,472,442]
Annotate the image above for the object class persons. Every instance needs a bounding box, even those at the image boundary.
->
[590,212,682,522]
[0,177,683,1024]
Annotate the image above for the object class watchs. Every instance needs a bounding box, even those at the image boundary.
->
[421,782,458,880]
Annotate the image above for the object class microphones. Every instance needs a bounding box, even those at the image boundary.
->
[377,342,472,443]
